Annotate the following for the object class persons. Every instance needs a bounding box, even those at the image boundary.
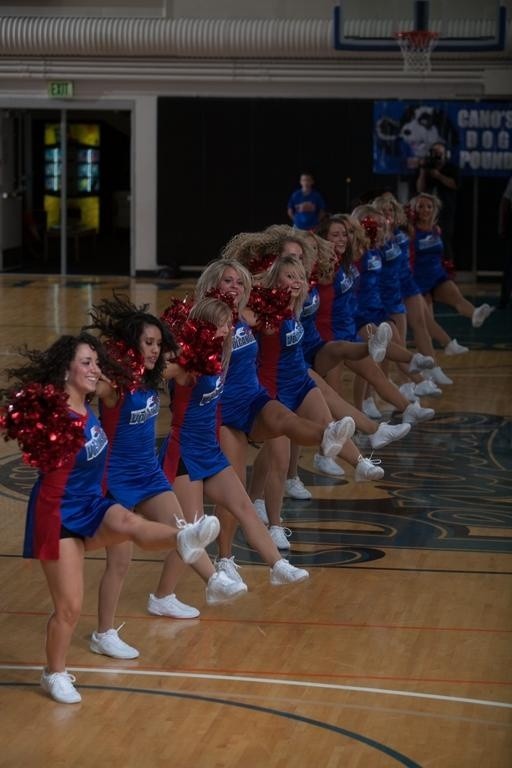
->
[223,233,411,500]
[362,195,470,418]
[266,224,394,476]
[146,298,310,619]
[89,313,201,660]
[193,259,355,582]
[410,192,490,330]
[19,334,219,703]
[261,257,385,550]
[351,203,445,414]
[285,172,323,231]
[320,218,436,426]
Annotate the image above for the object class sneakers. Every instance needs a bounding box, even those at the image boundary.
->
[472,304,490,329]
[284,477,312,499]
[268,525,292,551]
[147,593,201,619]
[252,498,283,525]
[313,454,345,475]
[399,382,420,402]
[321,415,355,456]
[365,322,393,363]
[444,339,469,356]
[401,401,435,427]
[354,453,384,484]
[90,628,140,660]
[414,377,442,396]
[176,513,221,565]
[205,570,247,604]
[268,558,310,587]
[39,667,82,705]
[214,554,242,582]
[363,396,382,419]
[431,366,453,385]
[367,419,411,450]
[408,353,434,375]
